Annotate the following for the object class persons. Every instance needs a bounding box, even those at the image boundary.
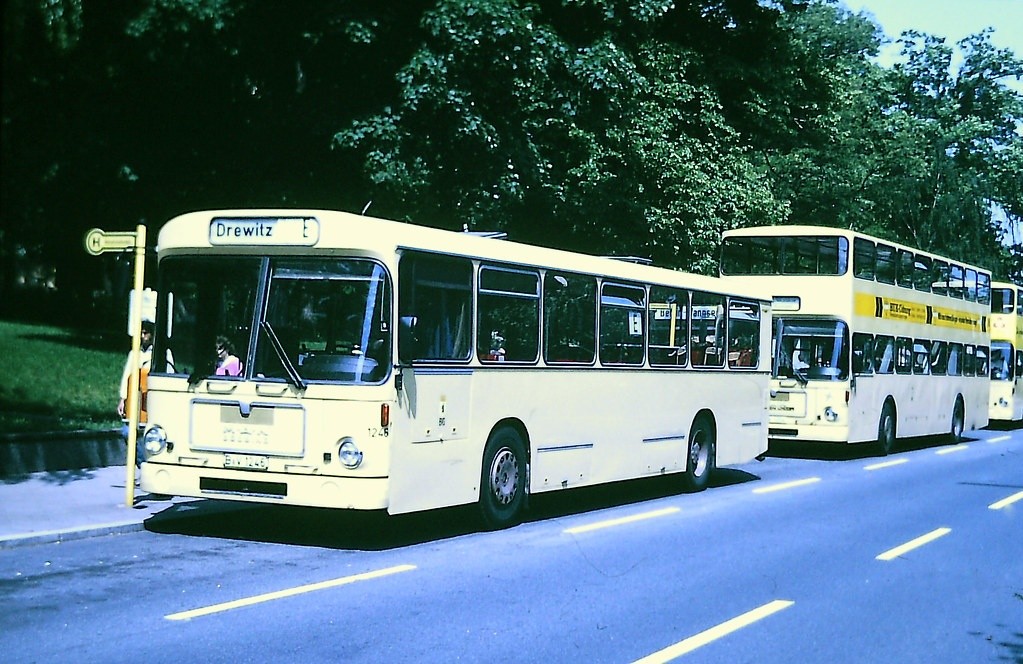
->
[489,329,505,361]
[213,336,245,378]
[116,318,175,489]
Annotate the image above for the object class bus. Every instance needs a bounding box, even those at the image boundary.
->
[927,282,1023,425]
[137,205,774,532]
[717,224,993,455]
